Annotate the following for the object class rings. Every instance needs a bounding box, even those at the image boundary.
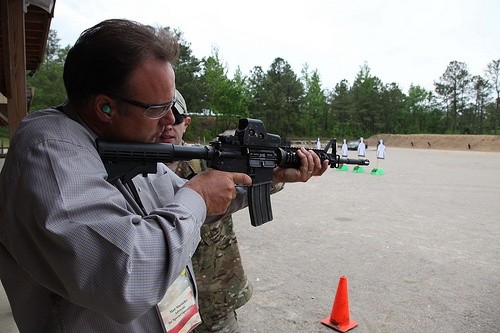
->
[308,170,312,174]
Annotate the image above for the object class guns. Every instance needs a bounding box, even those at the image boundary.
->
[96,117,370,227]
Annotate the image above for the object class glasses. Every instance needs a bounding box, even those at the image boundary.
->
[112,95,173,120]
[173,115,183,126]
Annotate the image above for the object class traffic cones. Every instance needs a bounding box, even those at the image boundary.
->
[320,275,359,332]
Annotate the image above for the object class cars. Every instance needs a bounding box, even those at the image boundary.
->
[347,140,368,150]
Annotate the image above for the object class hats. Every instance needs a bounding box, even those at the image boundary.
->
[173,88,190,116]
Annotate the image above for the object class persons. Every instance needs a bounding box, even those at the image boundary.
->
[0,19,329,333]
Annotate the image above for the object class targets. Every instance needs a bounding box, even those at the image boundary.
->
[315,136,387,160]
